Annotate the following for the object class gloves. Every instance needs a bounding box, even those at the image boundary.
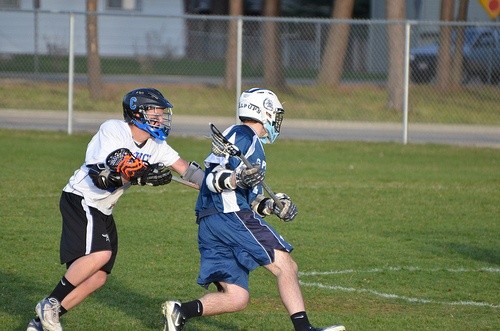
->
[131,162,172,187]
[264,193,298,222]
[235,164,266,189]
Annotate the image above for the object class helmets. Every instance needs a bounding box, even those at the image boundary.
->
[121,88,173,141]
[236,87,285,145]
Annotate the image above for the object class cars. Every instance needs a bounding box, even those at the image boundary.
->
[410,28,500,86]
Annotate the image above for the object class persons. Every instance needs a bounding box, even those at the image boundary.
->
[26,88,205,331]
[160,87,347,331]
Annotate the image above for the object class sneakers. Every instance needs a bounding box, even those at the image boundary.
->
[161,299,184,331]
[309,325,346,331]
[26,297,63,331]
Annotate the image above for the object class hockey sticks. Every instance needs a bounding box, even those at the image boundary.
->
[106,148,200,191]
[210,124,284,210]
